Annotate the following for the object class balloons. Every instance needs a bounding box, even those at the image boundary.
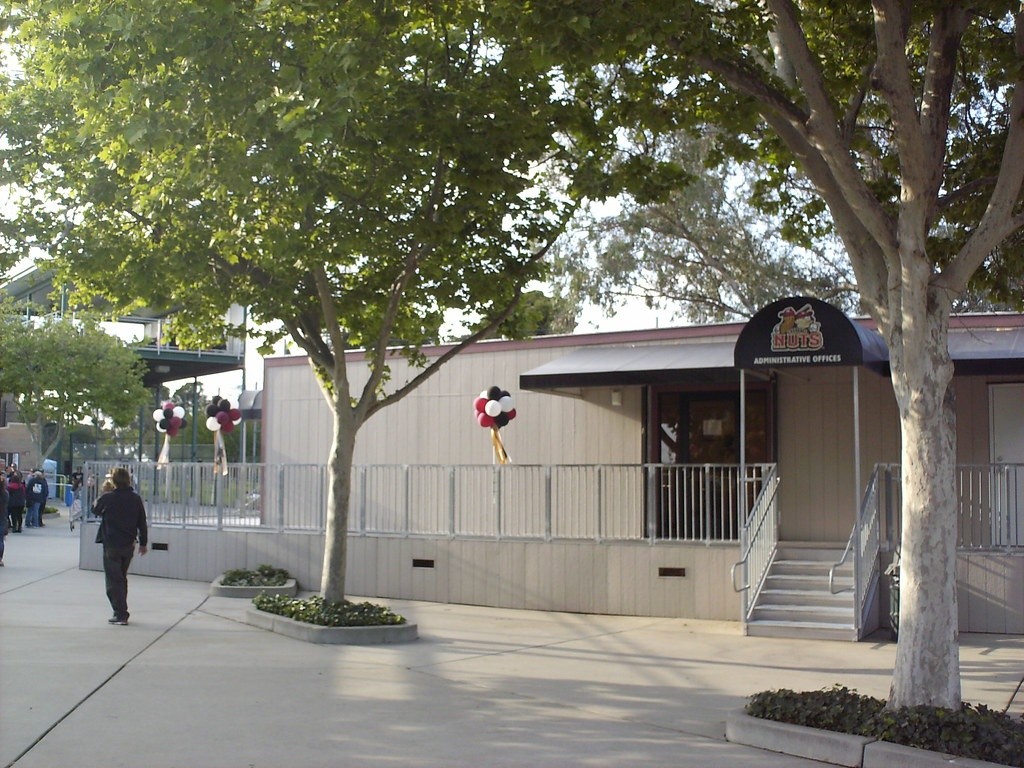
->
[205,396,243,432]
[153,401,188,436]
[473,387,518,429]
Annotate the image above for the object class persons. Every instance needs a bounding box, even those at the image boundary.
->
[91,464,147,625]
[0,458,50,567]
[69,467,97,521]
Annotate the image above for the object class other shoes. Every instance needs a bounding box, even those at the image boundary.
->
[24,524,32,528]
[108,616,129,625]
[39,524,45,527]
[0,558,4,565]
[32,525,39,528]
[12,529,16,533]
[18,530,21,533]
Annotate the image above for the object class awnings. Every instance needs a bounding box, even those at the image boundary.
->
[734,297,889,629]
[947,327,1024,360]
[519,342,737,399]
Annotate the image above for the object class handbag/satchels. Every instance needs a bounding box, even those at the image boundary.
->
[33,483,41,493]
